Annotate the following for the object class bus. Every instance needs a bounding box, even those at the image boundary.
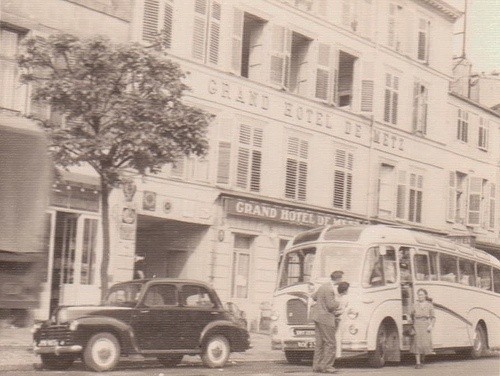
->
[270,224,500,367]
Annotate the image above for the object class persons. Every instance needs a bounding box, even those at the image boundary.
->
[313,272,343,373]
[409,289,436,369]
[334,282,350,369]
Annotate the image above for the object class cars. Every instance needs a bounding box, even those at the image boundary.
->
[33,278,252,371]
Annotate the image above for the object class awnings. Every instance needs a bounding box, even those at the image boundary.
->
[58,173,102,192]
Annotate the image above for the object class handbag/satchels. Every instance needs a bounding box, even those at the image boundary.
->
[402,325,416,335]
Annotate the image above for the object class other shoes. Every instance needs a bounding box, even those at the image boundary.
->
[415,364,421,368]
[313,369,322,373]
[322,369,335,373]
